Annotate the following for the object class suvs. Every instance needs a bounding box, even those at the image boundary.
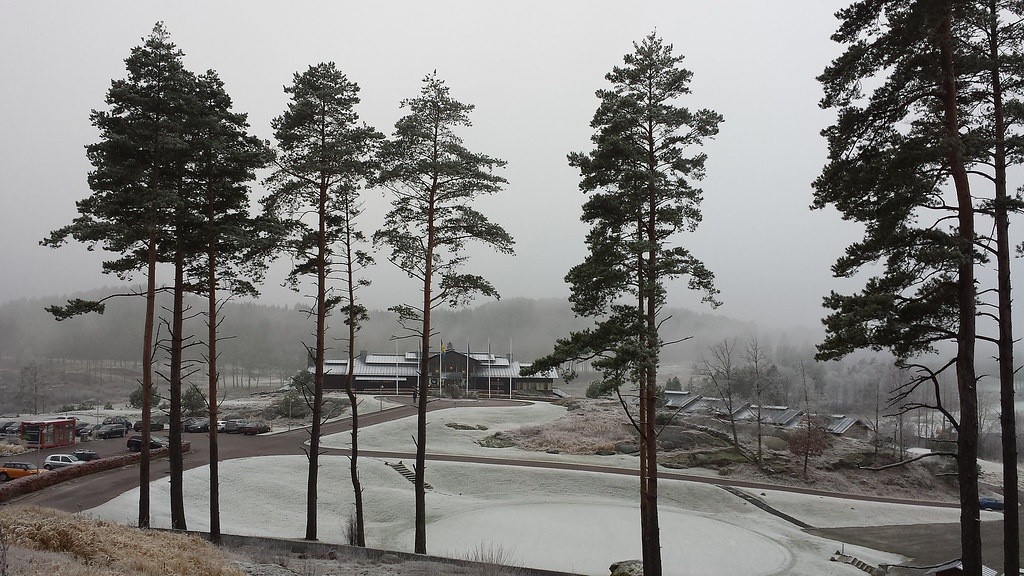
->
[128,434,169,452]
[103,416,132,430]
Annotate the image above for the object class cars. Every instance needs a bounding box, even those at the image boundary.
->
[0,461,50,482]
[98,424,128,439]
[0,421,22,435]
[188,419,210,432]
[44,453,86,471]
[133,419,164,432]
[243,421,271,434]
[980,496,1004,510]
[225,419,246,432]
[71,449,101,461]
[57,416,89,437]
[79,423,100,437]
[208,421,227,432]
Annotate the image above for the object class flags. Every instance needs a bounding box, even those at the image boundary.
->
[440,338,445,353]
[419,339,422,354]
[468,342,470,357]
[489,342,490,358]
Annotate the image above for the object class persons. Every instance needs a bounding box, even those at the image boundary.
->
[413,389,417,403]
